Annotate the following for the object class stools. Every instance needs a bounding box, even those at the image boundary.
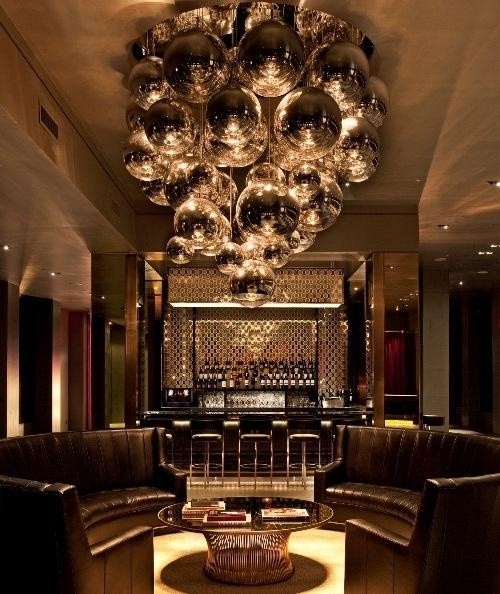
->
[329,418,364,463]
[411,414,446,432]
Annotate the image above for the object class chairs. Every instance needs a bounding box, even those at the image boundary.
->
[285,416,323,489]
[140,416,176,468]
[187,418,226,489]
[237,413,276,489]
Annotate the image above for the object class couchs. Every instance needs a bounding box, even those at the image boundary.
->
[1,428,190,593]
[316,422,500,592]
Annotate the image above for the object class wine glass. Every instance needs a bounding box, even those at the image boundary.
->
[198,390,316,408]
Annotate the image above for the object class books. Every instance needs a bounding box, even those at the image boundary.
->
[182,498,251,524]
[261,508,310,521]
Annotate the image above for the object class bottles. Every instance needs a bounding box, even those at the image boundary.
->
[321,387,354,406]
[196,360,316,389]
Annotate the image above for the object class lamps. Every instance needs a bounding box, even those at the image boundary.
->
[119,0,391,312]
